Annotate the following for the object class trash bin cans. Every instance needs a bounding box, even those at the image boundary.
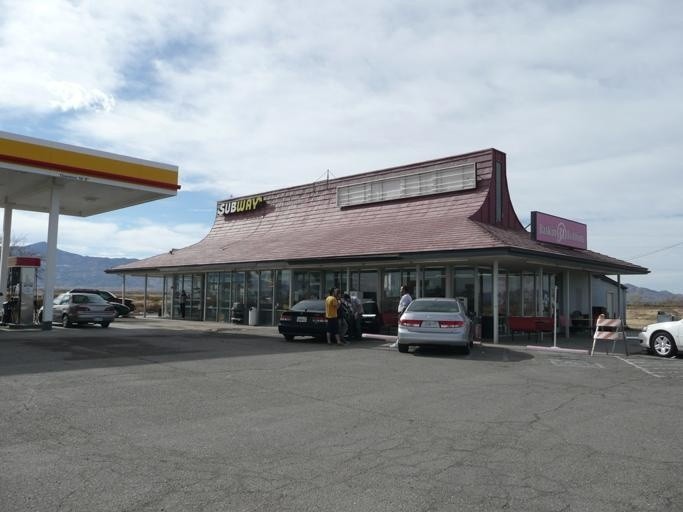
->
[231,302,244,323]
[249,307,257,326]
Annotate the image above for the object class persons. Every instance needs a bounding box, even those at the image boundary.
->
[343,294,364,341]
[396,285,412,318]
[178,289,187,319]
[324,287,343,346]
[333,288,348,344]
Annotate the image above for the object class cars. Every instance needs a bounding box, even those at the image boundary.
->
[396,297,476,356]
[278,299,366,344]
[637,319,682,358]
[36,289,136,329]
